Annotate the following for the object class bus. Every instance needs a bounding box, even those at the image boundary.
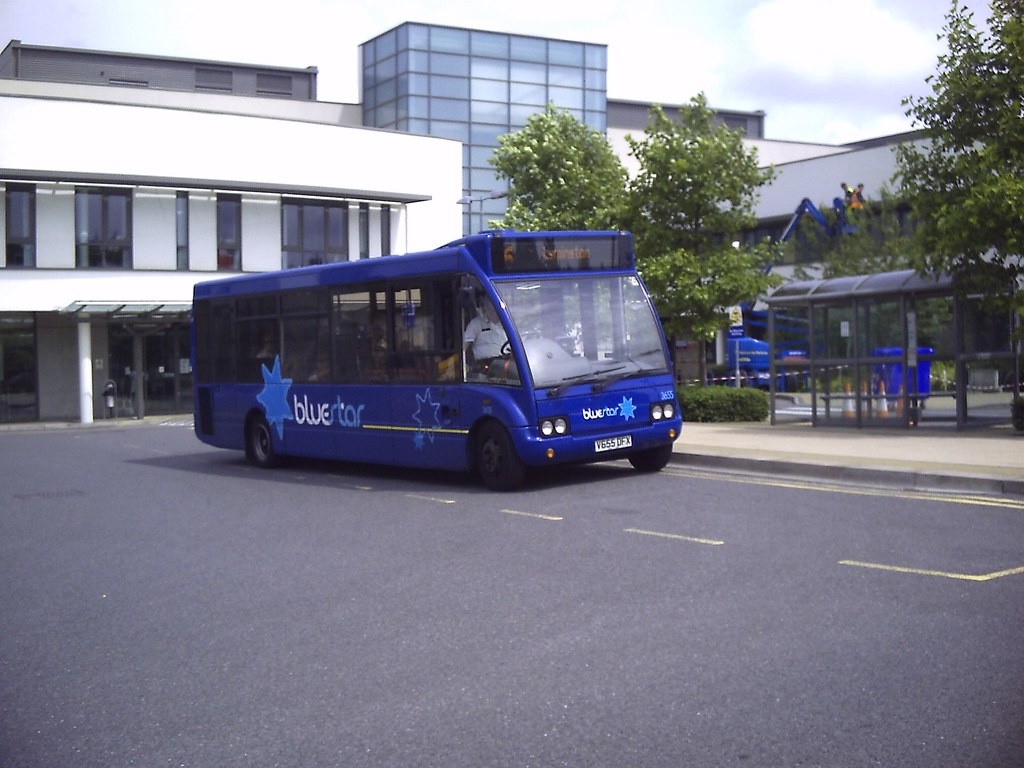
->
[191,229,683,491]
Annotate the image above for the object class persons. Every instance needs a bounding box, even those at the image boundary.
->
[463,288,512,372]
[366,325,387,350]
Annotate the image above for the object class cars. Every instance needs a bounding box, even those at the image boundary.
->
[0,373,37,414]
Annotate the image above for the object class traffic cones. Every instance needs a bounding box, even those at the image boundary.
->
[876,381,889,418]
[841,382,854,418]
[894,386,911,427]
[858,381,867,417]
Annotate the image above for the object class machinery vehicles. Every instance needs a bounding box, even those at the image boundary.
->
[661,196,861,387]
